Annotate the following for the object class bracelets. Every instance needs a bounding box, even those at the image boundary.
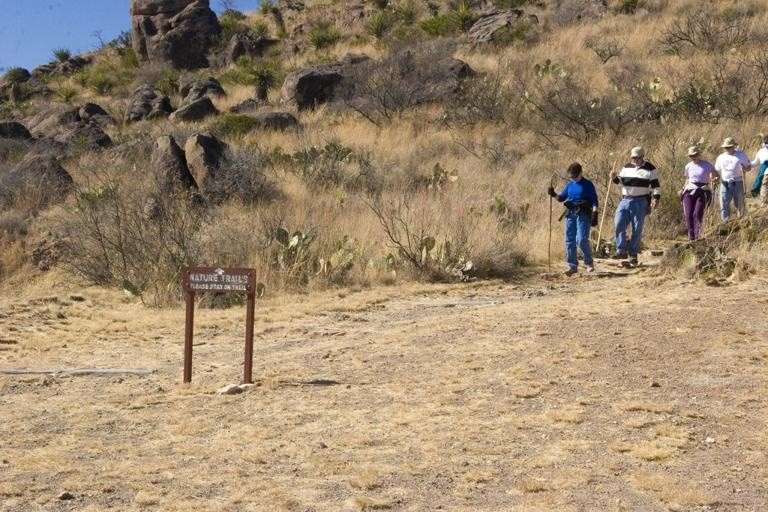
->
[682,189,684,191]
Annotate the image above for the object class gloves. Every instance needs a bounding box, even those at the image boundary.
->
[590,211,598,226]
[548,187,557,197]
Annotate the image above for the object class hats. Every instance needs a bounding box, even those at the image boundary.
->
[688,146,698,155]
[630,147,643,158]
[720,137,738,148]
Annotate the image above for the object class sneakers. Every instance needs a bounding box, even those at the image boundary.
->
[612,254,627,259]
[630,255,637,262]
[562,269,577,274]
[587,259,594,272]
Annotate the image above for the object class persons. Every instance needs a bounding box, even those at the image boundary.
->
[678,146,720,242]
[750,134,768,209]
[609,146,661,264]
[712,136,752,221]
[548,162,598,276]
